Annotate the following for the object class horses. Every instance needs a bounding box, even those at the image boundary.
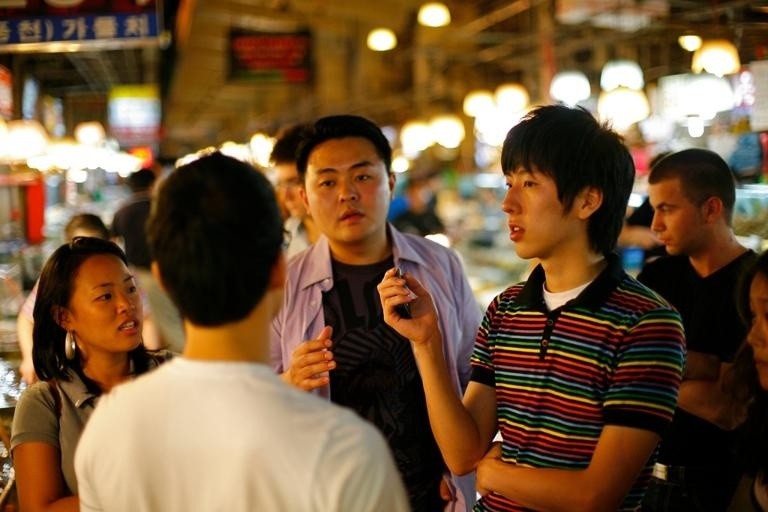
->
[395,266,413,320]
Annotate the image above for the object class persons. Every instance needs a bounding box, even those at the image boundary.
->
[633,147,763,512]
[263,118,314,261]
[375,96,687,512]
[111,169,171,354]
[15,211,161,387]
[69,147,416,512]
[616,147,672,271]
[7,235,182,512]
[392,177,447,235]
[266,112,482,512]
[741,246,766,397]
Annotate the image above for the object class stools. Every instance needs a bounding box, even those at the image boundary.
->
[652,463,697,483]
